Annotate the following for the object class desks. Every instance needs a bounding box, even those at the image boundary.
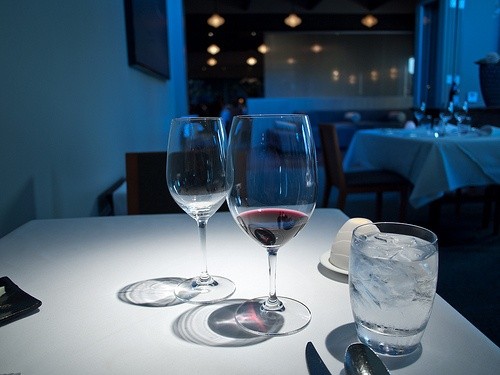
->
[0,209,500,375]
[358,122,500,228]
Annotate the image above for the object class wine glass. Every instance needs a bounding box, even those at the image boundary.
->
[166,117,236,304]
[224,113,319,337]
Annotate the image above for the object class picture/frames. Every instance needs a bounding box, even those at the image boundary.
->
[123,0,171,81]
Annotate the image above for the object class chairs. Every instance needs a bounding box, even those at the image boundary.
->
[97,151,230,215]
[316,124,415,223]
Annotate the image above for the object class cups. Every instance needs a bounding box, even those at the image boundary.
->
[349,221,439,357]
[330,218,381,271]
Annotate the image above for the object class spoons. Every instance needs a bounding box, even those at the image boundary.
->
[344,342,391,375]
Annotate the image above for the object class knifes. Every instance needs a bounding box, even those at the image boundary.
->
[305,341,333,375]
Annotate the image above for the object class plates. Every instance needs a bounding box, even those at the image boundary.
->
[320,250,349,275]
